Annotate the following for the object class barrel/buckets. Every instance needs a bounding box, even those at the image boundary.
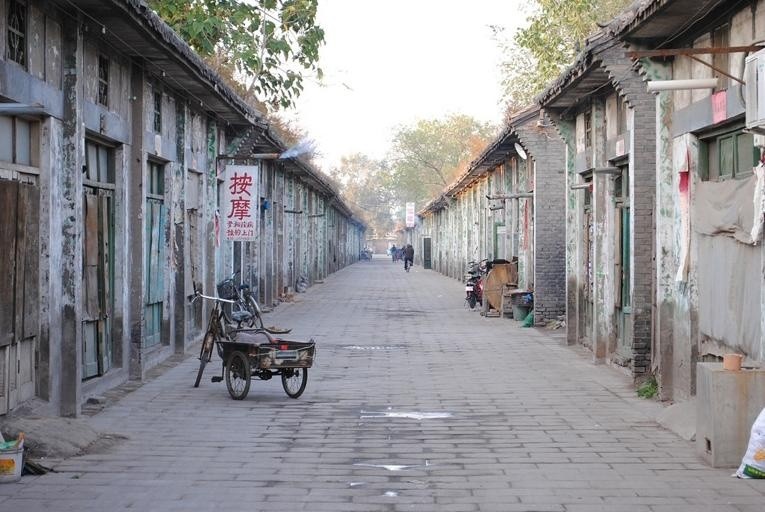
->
[0,447,24,484]
[512,293,527,322]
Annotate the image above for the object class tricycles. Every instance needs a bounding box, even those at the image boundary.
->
[186,289,318,403]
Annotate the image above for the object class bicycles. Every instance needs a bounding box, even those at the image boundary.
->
[404,257,412,273]
[217,267,265,330]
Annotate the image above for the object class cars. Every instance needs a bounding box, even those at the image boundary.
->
[386,249,392,257]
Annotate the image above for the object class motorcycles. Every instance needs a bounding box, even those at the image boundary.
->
[465,258,488,310]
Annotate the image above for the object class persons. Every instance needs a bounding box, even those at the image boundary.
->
[390,243,415,271]
